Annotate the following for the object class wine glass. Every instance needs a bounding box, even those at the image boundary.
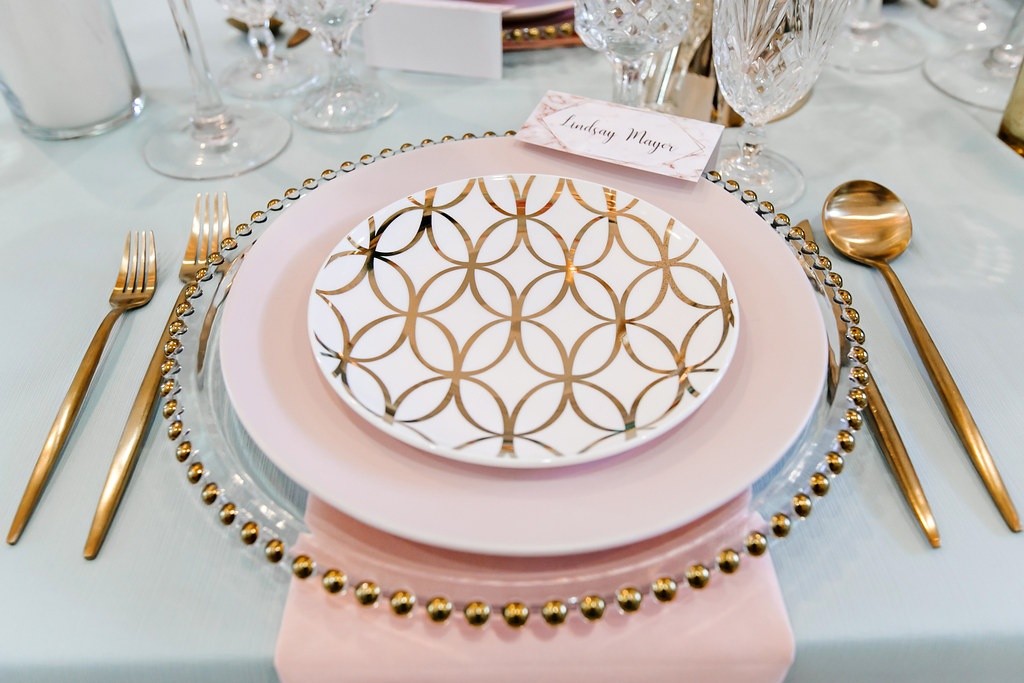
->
[921,0,1024,110]
[572,0,711,120]
[209,0,318,100]
[825,0,929,75]
[142,0,293,177]
[277,0,401,132]
[711,1,847,209]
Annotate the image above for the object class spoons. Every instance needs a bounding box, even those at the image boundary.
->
[820,179,1022,536]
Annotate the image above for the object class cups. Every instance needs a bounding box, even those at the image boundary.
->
[996,61,1024,156]
[0,0,144,142]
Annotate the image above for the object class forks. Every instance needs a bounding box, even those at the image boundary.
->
[5,230,158,541]
[83,193,231,556]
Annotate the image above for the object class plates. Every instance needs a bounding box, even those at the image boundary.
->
[501,20,575,43]
[162,131,868,623]
[305,168,742,471]
[218,134,828,555]
[492,0,573,20]
